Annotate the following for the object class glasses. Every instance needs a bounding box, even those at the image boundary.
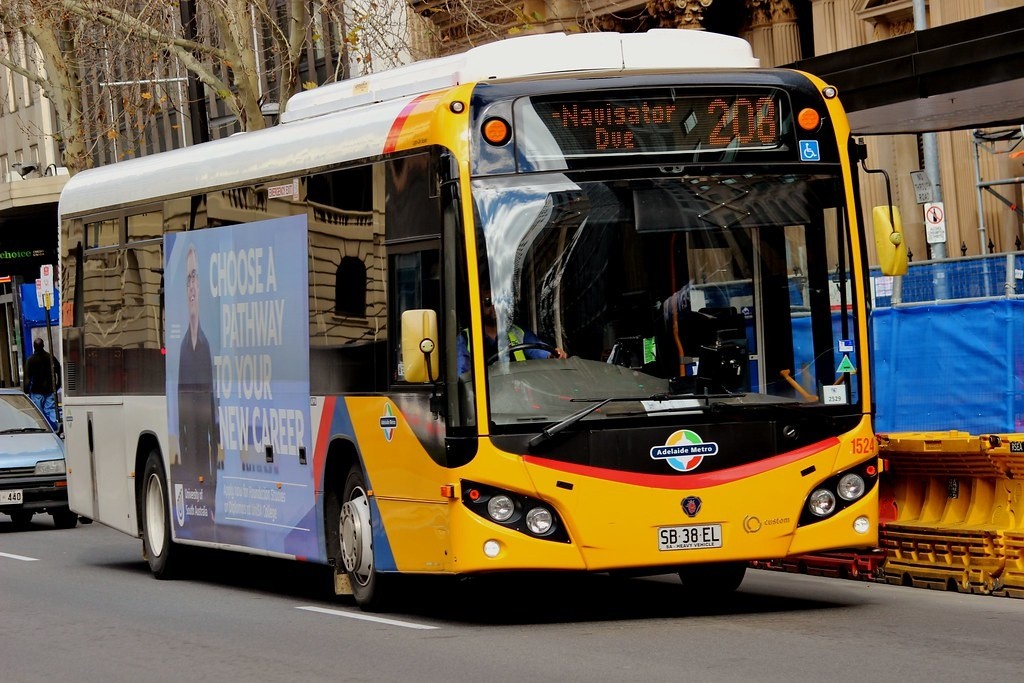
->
[482,297,512,307]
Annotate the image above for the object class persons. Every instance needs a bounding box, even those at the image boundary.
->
[455,276,568,380]
[179,247,221,541]
[23,338,61,432]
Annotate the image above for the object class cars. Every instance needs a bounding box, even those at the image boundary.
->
[0,388,79,531]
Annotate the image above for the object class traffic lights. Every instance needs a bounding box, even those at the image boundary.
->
[159,343,166,358]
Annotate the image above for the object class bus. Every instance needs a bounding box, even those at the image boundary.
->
[57,27,909,612]
